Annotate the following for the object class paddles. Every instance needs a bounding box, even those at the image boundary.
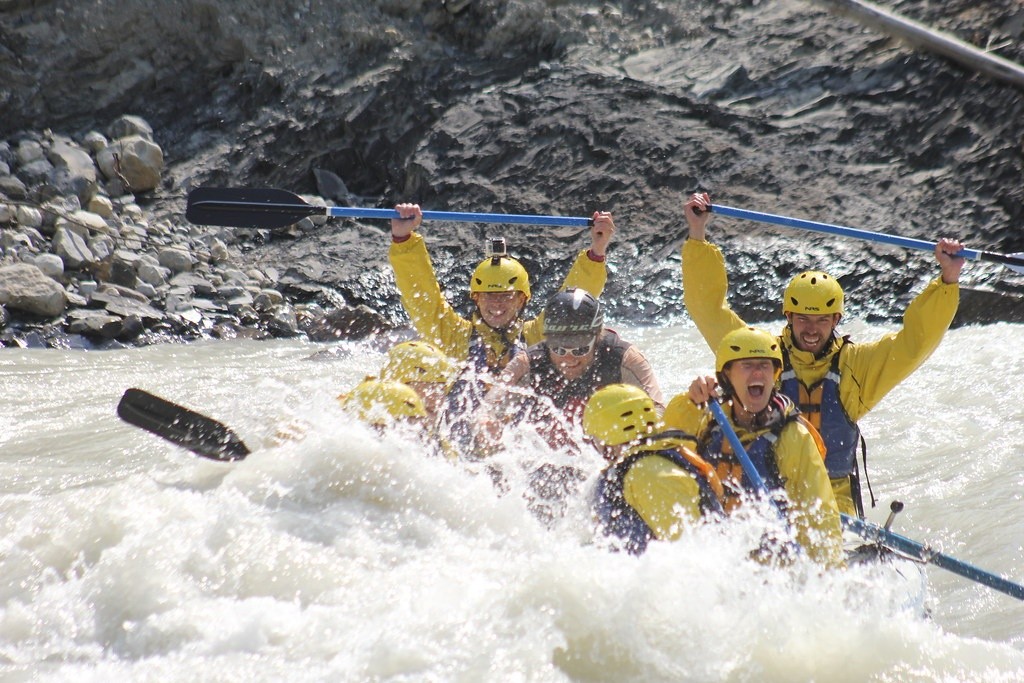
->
[832,512,1024,601]
[115,386,251,464]
[692,203,1024,276]
[181,185,598,228]
[699,382,805,558]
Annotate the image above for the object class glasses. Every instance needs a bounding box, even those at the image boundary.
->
[548,335,596,357]
[478,292,516,304]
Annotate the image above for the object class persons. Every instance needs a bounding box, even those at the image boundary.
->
[389,202,615,376]
[347,343,461,457]
[470,287,659,418]
[582,384,726,558]
[682,191,966,520]
[662,329,843,569]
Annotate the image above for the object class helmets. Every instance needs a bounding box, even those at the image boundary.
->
[782,271,844,326]
[583,383,658,446]
[354,381,426,424]
[542,286,603,343]
[714,326,784,389]
[470,257,530,310]
[380,341,457,382]
